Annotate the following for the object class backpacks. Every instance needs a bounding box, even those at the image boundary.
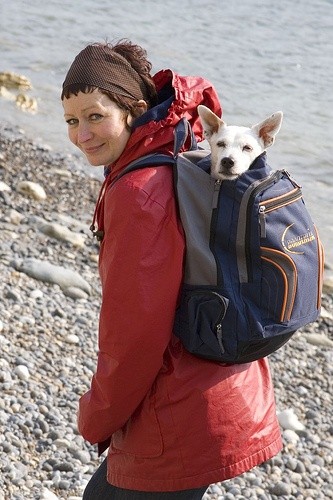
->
[108,117,324,365]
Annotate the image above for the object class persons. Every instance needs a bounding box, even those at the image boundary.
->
[60,37,284,500]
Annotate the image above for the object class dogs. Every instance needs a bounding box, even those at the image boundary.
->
[196,104,283,181]
